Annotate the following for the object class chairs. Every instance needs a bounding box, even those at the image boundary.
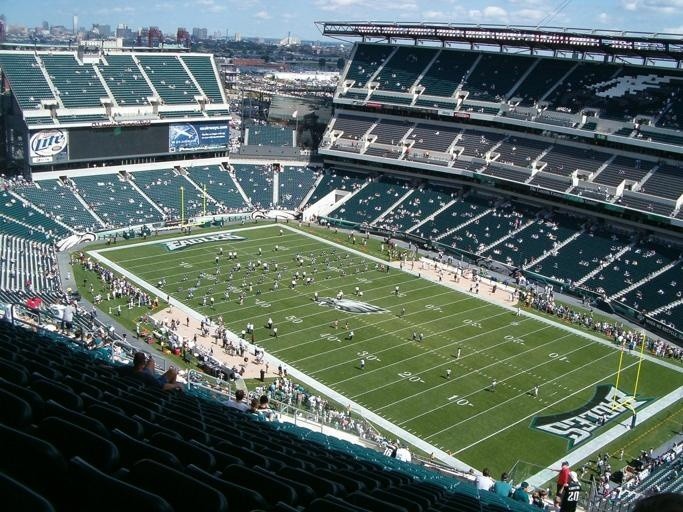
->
[2,313,555,512]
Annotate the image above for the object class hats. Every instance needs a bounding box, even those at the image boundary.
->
[569,471,578,482]
[521,482,528,488]
